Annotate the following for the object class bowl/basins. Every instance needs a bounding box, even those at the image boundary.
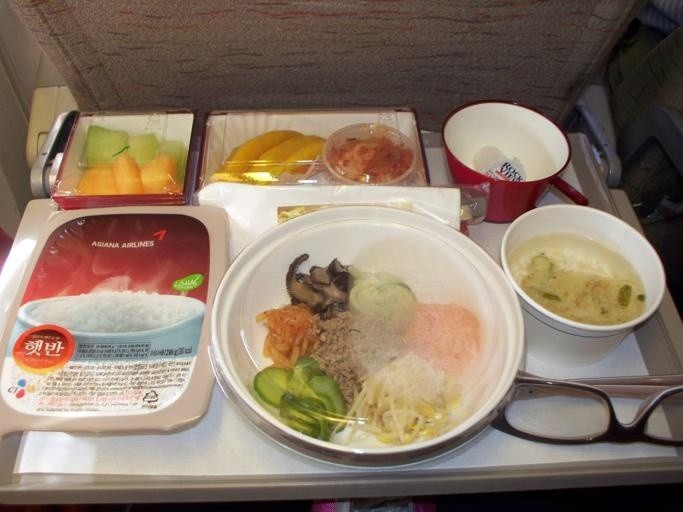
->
[500,204,669,380]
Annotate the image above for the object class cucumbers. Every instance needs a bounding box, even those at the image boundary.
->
[253,356,347,441]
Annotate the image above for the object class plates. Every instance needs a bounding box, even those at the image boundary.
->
[210,205,529,469]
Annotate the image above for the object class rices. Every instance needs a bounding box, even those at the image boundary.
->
[31,290,195,332]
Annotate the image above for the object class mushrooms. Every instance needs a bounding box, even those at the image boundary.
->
[286,254,355,320]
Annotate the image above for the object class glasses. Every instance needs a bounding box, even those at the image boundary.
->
[490,369,683,447]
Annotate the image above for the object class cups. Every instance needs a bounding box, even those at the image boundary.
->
[442,97,589,224]
